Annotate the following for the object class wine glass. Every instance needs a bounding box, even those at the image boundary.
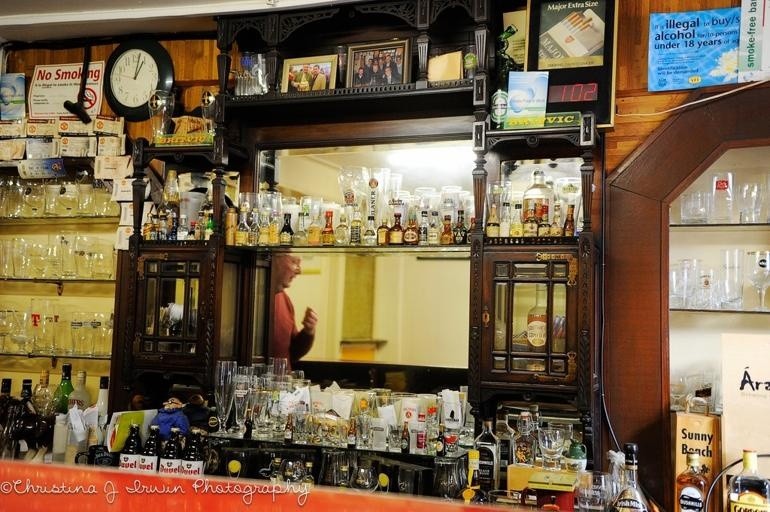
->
[669,371,717,416]
[750,251,769,314]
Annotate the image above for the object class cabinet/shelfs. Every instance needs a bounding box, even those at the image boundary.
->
[0,156,117,363]
[130,251,211,384]
[670,222,768,315]
[471,248,591,415]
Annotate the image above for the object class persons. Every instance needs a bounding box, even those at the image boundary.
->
[288,48,403,91]
[267,253,319,376]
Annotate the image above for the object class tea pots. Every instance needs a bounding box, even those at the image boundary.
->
[564,438,589,472]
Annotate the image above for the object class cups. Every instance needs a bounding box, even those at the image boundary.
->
[708,171,734,225]
[74,445,113,467]
[740,181,764,224]
[576,470,610,512]
[213,358,475,453]
[0,178,120,220]
[271,453,479,498]
[0,295,116,356]
[486,489,522,511]
[547,422,574,455]
[232,49,274,97]
[0,229,116,280]
[148,89,174,144]
[680,192,713,225]
[719,249,746,309]
[539,426,563,470]
[672,258,714,308]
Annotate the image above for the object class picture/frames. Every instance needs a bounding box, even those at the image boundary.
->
[281,53,337,92]
[344,36,414,89]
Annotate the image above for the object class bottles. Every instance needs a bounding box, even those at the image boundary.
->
[477,406,543,498]
[141,169,215,242]
[550,315,566,353]
[608,443,665,512]
[526,282,551,352]
[489,64,510,131]
[225,165,580,247]
[0,364,109,461]
[673,451,714,512]
[725,450,770,512]
[115,422,250,478]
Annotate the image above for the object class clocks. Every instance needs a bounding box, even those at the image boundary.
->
[103,41,174,120]
